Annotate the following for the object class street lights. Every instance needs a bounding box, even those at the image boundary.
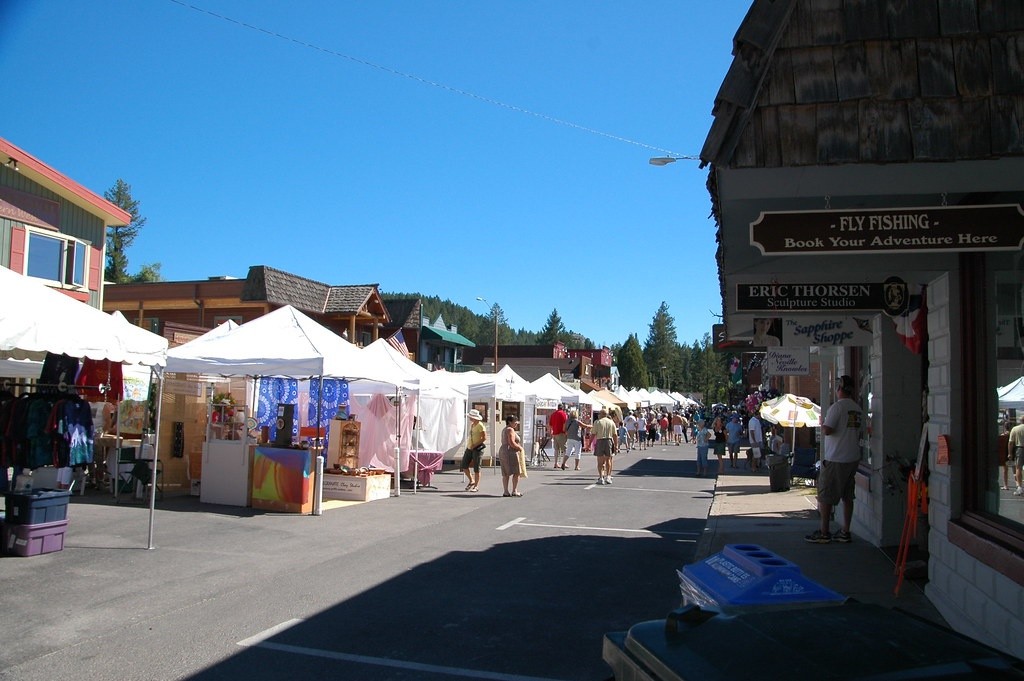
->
[476,297,499,422]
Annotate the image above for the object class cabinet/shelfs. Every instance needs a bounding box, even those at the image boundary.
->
[323,472,391,501]
[251,445,321,514]
[326,420,361,470]
[200,441,259,509]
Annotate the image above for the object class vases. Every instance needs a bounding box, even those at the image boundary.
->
[913,498,930,545]
[212,406,234,424]
[746,448,762,470]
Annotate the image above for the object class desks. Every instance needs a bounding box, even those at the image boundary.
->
[399,450,444,489]
[94,433,123,497]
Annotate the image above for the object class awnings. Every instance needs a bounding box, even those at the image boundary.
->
[422,326,475,347]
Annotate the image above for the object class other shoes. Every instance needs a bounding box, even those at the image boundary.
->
[565,464,569,467]
[596,479,604,484]
[561,464,565,470]
[606,477,612,484]
[1013,487,1022,496]
[1001,487,1009,490]
[470,487,478,492]
[511,492,523,498]
[575,468,580,470]
[554,464,561,468]
[465,482,475,490]
[503,493,512,497]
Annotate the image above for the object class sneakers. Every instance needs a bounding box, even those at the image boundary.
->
[831,529,851,543]
[803,530,832,543]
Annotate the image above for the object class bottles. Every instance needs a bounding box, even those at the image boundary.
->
[236,410,244,422]
[298,436,310,450]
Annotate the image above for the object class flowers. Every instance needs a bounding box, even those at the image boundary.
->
[744,390,784,418]
[895,458,930,506]
[211,392,237,411]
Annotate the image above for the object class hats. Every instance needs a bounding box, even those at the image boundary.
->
[467,409,483,420]
[628,411,634,413]
[835,375,855,392]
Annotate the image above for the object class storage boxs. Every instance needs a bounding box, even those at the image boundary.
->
[0,489,72,557]
[13,474,32,492]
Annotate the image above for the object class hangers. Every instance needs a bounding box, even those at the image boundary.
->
[0,381,93,408]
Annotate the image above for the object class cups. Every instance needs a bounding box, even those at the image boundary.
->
[261,426,269,443]
[350,413,358,420]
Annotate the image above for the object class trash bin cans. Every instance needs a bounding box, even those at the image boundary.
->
[674,545,848,612]
[603,596,1024,680]
[765,454,793,492]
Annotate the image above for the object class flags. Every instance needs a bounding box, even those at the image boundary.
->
[389,330,409,357]
[730,357,740,373]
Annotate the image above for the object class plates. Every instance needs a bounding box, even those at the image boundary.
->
[360,467,368,472]
[247,418,257,429]
[340,465,351,473]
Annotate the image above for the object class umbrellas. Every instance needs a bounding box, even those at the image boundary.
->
[759,393,821,466]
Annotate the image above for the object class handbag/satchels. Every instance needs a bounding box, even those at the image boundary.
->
[752,444,762,458]
[475,443,485,451]
[656,432,661,441]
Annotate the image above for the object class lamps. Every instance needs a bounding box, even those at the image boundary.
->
[649,157,704,166]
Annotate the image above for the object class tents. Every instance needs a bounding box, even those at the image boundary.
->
[0,265,168,548]
[997,376,1024,408]
[140,304,699,512]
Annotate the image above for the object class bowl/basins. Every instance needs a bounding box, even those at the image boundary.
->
[369,468,385,474]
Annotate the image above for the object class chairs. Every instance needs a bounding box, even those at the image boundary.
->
[790,447,818,488]
[114,459,161,505]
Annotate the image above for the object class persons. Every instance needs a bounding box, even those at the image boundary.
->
[461,410,487,492]
[588,409,618,484]
[753,318,780,347]
[803,375,866,544]
[499,415,524,497]
[548,403,569,468]
[607,405,763,478]
[561,411,593,471]
[1000,415,1024,495]
